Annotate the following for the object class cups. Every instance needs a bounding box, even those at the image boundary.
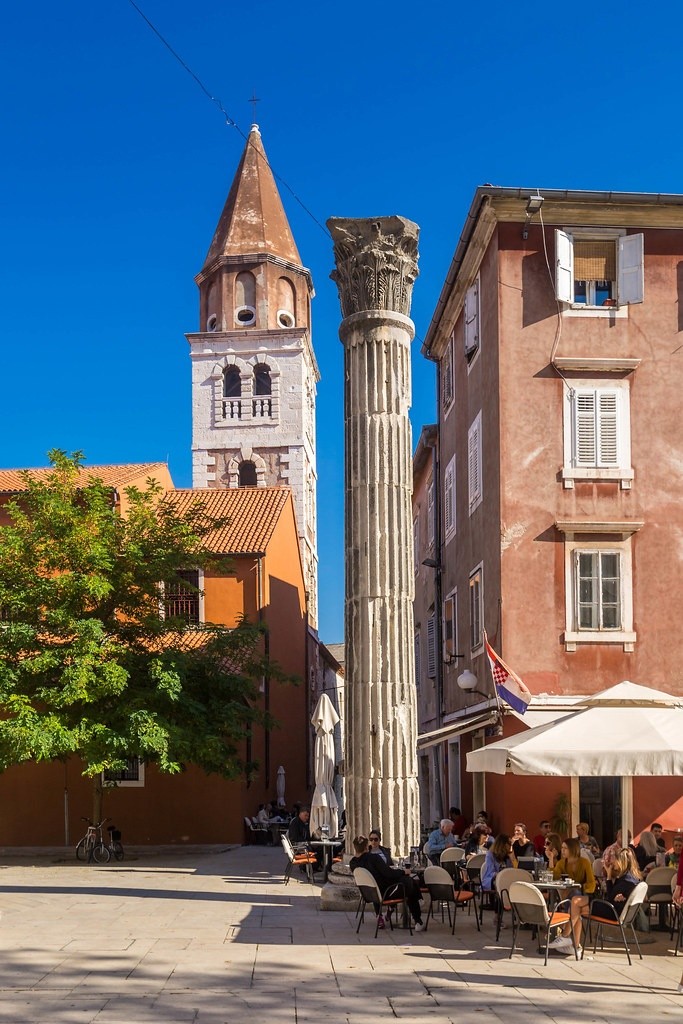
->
[547,874,553,884]
[542,871,552,882]
[399,858,404,868]
[405,864,411,869]
[565,879,572,884]
[561,874,569,881]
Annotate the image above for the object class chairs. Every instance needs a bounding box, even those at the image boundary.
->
[281,830,345,886]
[353,846,683,966]
[245,816,273,845]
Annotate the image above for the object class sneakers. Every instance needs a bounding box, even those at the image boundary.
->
[555,944,583,955]
[540,936,572,948]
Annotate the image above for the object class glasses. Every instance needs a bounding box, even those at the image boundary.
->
[369,838,379,842]
[543,825,552,829]
[543,841,551,846]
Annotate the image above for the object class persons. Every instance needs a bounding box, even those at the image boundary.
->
[450,807,683,954]
[369,831,394,928]
[289,807,323,875]
[349,835,425,931]
[429,819,457,866]
[257,803,272,829]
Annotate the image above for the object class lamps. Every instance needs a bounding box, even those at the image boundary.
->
[422,558,442,575]
[523,196,545,240]
[448,654,465,665]
[457,669,491,700]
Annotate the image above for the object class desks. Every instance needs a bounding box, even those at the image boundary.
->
[528,882,581,956]
[390,866,424,929]
[271,822,290,823]
[311,841,342,883]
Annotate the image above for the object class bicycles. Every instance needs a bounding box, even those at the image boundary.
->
[93,818,124,863]
[75,816,96,864]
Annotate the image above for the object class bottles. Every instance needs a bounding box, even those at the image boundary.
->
[534,857,544,881]
[655,850,665,867]
[419,851,423,865]
[669,860,673,867]
[414,851,418,866]
[410,848,414,865]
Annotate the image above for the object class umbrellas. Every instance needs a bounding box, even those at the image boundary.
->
[277,766,286,808]
[310,693,341,839]
[465,681,683,776]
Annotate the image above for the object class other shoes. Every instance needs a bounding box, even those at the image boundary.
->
[384,907,394,921]
[376,915,385,929]
[414,922,425,931]
[493,917,505,929]
[513,922,526,930]
[399,918,415,928]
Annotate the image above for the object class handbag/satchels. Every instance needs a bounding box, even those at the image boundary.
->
[637,906,649,931]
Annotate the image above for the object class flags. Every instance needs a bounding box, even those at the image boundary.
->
[486,642,532,714]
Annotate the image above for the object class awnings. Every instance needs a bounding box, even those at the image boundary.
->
[418,711,498,750]
